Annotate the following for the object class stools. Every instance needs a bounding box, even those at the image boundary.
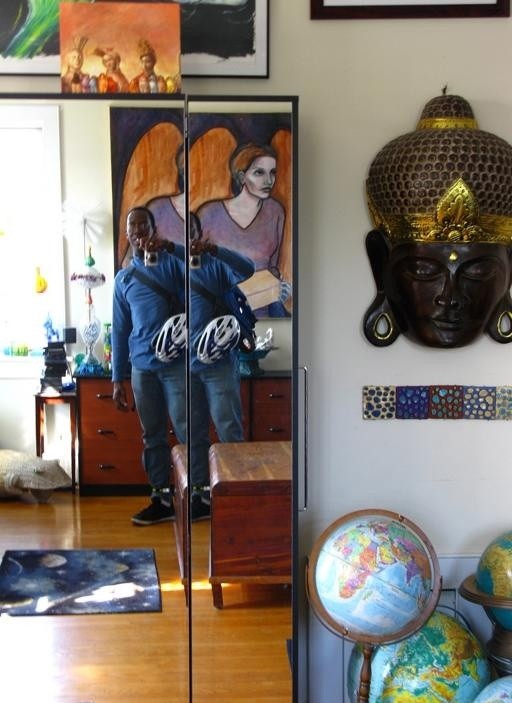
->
[169,443,190,610]
[210,442,297,609]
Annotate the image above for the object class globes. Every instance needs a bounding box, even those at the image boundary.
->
[305,511,446,703]
[458,529,512,674]
[347,603,488,703]
[473,669,512,703]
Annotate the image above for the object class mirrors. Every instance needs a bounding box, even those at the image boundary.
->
[189,95,296,701]
[1,93,191,703]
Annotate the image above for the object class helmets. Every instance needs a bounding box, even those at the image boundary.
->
[195,313,242,365]
[152,311,189,364]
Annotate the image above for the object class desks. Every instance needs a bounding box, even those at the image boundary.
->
[32,391,78,492]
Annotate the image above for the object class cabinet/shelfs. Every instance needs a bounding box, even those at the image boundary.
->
[74,373,178,495]
[207,369,289,482]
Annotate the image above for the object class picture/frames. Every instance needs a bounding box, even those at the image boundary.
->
[308,0,511,20]
[0,0,270,80]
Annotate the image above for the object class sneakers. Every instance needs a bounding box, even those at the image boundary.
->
[130,495,177,526]
[189,493,212,522]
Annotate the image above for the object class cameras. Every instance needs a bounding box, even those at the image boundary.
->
[143,242,159,267]
[190,243,200,268]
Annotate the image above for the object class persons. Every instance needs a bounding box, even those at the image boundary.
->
[122,144,187,278]
[112,204,190,525]
[126,39,164,91]
[63,47,90,94]
[194,141,290,317]
[359,83,511,349]
[184,209,257,522]
[96,45,128,91]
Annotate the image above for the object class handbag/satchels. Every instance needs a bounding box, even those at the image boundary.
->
[212,295,258,354]
